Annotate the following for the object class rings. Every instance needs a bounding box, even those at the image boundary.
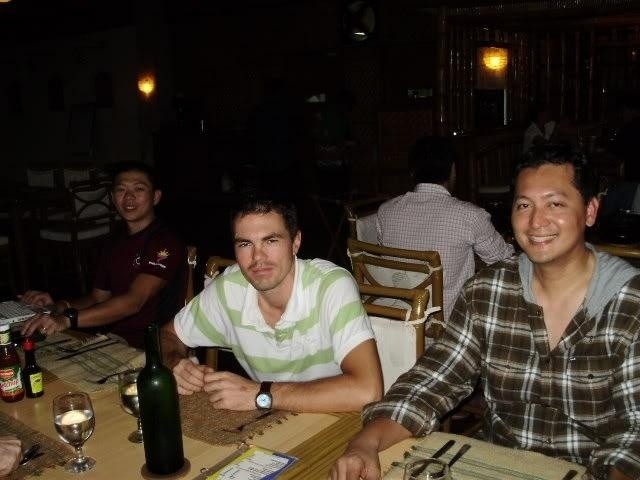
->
[41,327,47,331]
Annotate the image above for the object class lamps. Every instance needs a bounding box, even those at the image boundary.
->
[477,29,513,72]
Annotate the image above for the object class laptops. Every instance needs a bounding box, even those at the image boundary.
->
[0,298,35,331]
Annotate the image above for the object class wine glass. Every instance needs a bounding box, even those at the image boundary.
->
[50,389,100,476]
[119,366,150,445]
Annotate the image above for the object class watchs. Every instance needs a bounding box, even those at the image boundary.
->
[63,307,78,331]
[255,381,274,412]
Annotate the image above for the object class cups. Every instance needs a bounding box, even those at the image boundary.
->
[402,457,453,480]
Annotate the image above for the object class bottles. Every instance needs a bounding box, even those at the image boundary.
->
[0,322,45,404]
[137,322,192,474]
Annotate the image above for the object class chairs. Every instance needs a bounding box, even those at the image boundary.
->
[0,175,123,302]
[359,285,430,396]
[347,237,445,341]
[203,255,238,371]
[182,244,198,307]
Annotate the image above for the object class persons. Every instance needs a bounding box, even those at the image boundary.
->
[1,434,23,477]
[158,190,385,414]
[327,144,639,480]
[376,136,515,352]
[17,161,190,350]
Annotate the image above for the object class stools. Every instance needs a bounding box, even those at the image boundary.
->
[309,192,388,264]
